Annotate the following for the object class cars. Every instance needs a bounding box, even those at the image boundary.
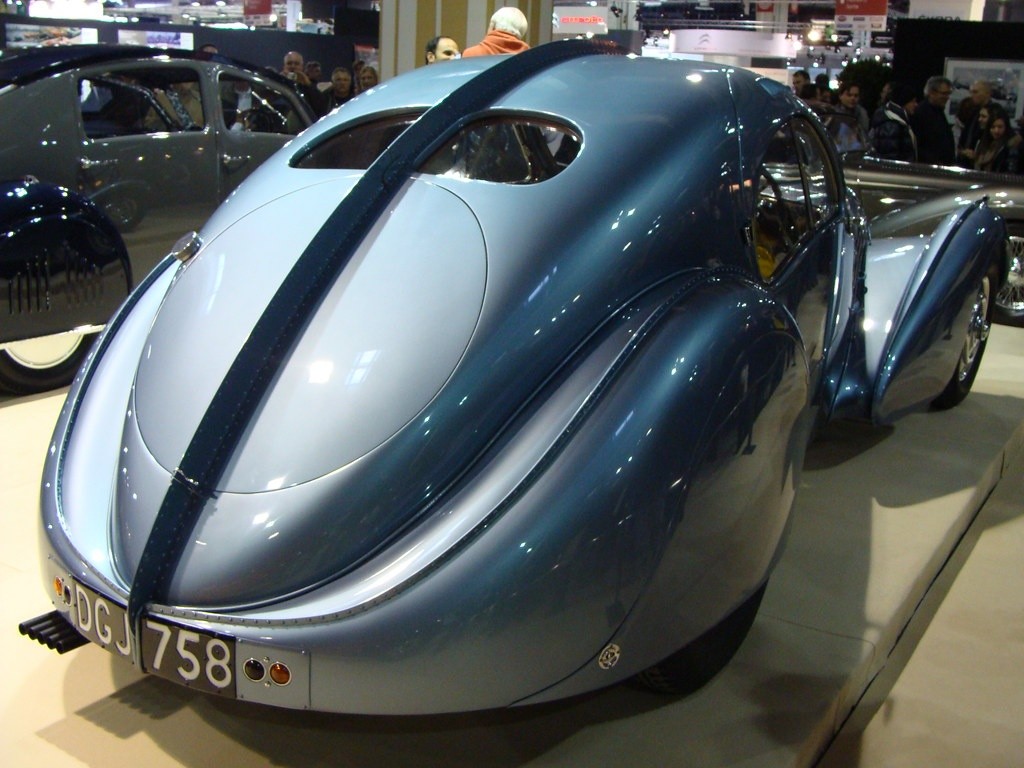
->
[34,30,1012,719]
[0,47,344,393]
[759,100,1024,328]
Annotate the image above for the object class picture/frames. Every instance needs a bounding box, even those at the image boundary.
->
[943,57,1024,131]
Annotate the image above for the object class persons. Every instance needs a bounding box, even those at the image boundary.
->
[461,7,531,57]
[198,44,218,53]
[221,60,255,132]
[144,81,205,130]
[425,36,459,64]
[279,51,378,113]
[791,71,1024,174]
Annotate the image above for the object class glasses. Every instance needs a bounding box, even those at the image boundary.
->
[932,90,952,95]
